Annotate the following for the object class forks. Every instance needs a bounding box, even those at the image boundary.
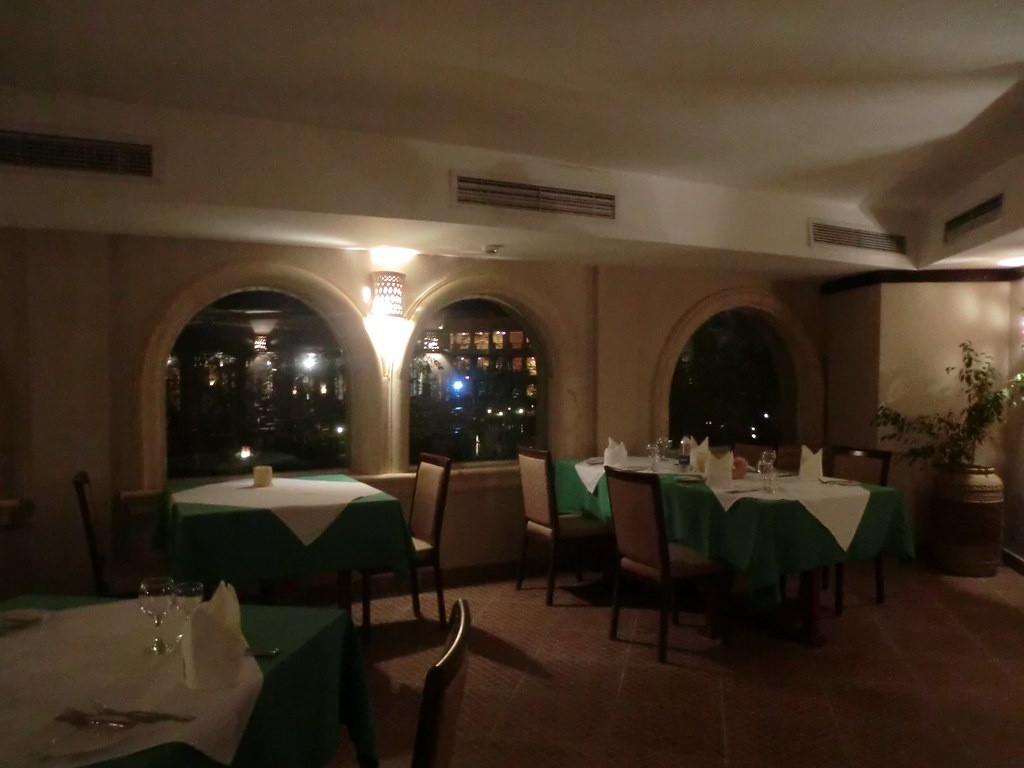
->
[93,702,196,723]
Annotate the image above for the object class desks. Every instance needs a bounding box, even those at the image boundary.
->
[551,454,915,647]
[166,473,418,637]
[0,590,347,768]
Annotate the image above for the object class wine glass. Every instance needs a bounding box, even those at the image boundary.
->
[756,449,778,494]
[136,576,174,656]
[645,437,672,473]
[174,581,204,645]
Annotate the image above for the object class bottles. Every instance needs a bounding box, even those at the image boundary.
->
[679,437,691,473]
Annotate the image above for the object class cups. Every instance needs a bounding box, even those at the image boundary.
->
[252,465,273,488]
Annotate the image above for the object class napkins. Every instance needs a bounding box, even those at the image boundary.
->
[603,436,627,469]
[798,444,823,480]
[184,580,247,689]
[690,436,710,473]
[704,451,734,490]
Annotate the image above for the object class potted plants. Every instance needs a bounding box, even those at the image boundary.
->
[870,340,1024,577]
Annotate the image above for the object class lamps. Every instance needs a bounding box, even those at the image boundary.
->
[370,271,405,318]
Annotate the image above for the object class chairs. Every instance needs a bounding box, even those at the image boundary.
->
[410,598,471,768]
[515,446,611,606]
[779,446,892,617]
[348,453,451,645]
[602,465,734,662]
[71,471,170,598]
